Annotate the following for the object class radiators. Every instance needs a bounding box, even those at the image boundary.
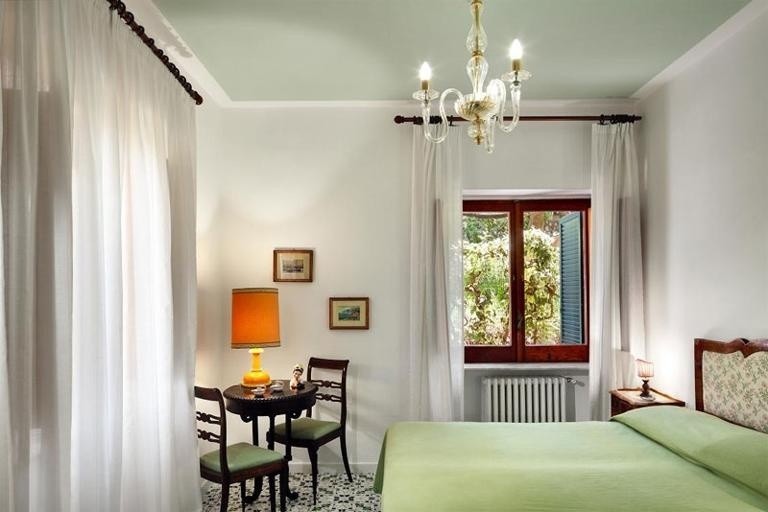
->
[481,376,576,423]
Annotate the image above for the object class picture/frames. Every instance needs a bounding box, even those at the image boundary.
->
[329,296,369,330]
[273,249,313,282]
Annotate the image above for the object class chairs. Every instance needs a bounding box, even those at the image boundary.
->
[266,357,353,505]
[194,386,288,512]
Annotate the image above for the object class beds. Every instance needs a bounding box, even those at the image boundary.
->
[373,339,768,512]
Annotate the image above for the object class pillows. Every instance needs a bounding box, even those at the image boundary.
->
[613,405,768,498]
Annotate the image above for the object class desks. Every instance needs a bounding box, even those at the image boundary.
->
[223,379,318,503]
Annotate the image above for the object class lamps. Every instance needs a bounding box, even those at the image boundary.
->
[411,0,531,154]
[231,288,281,388]
[637,358,655,397]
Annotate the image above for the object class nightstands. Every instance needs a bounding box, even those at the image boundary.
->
[609,387,685,418]
